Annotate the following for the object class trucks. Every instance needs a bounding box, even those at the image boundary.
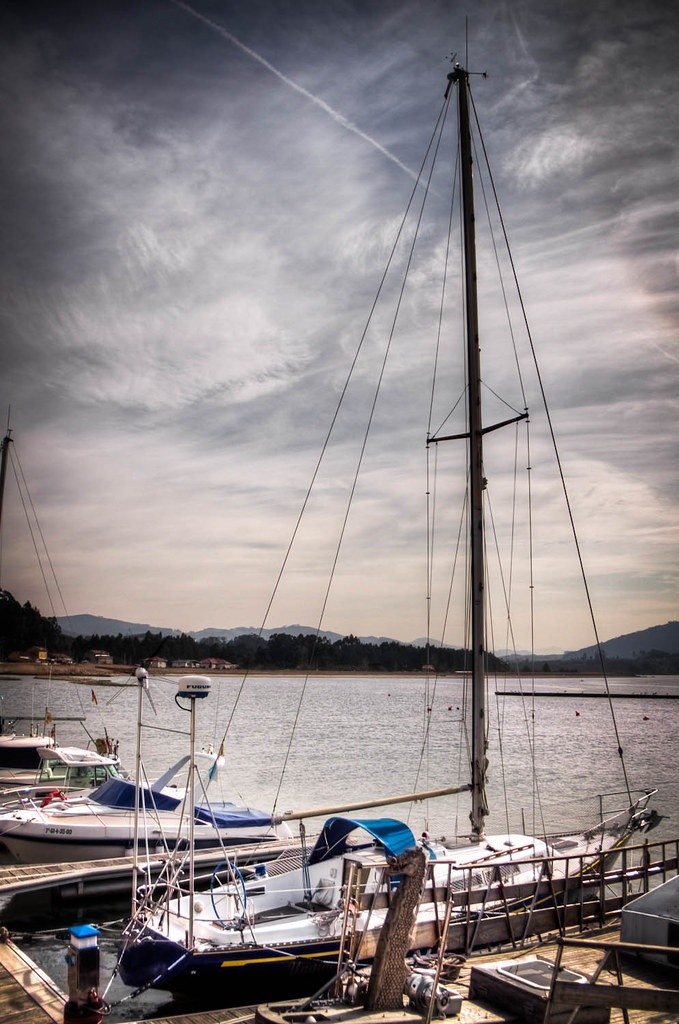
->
[62,658,73,665]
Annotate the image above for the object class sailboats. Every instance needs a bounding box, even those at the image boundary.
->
[130,59,662,992]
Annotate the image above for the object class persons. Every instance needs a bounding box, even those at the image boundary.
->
[108,738,114,754]
[8,721,14,731]
[114,740,119,755]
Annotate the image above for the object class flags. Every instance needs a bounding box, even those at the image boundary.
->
[51,727,55,736]
[210,763,217,783]
[45,708,51,723]
[91,689,97,705]
[218,744,224,758]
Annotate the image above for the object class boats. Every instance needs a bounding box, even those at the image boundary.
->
[1,745,125,805]
[0,750,293,864]
[0,733,58,768]
